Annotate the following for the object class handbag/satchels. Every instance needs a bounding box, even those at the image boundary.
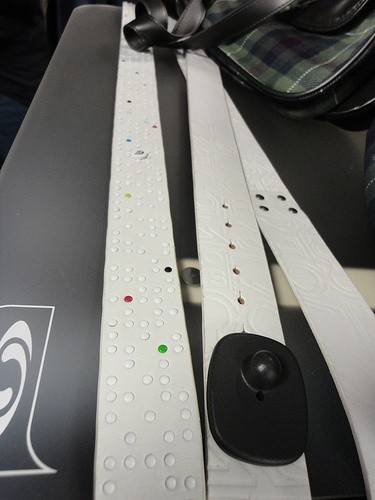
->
[122,1,375,133]
